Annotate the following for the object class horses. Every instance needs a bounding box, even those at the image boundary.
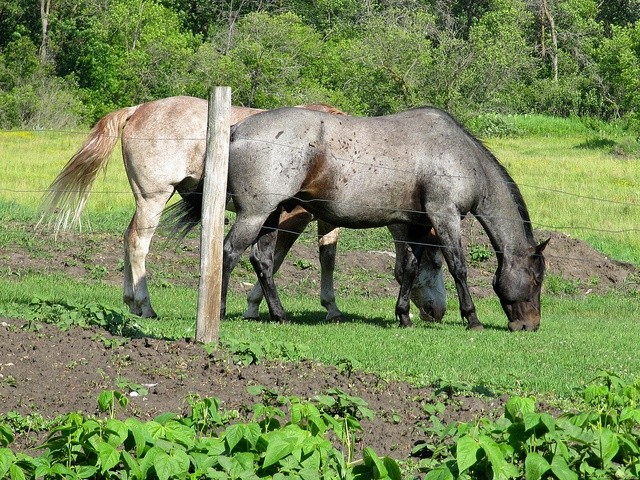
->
[33,96,446,321]
[154,106,551,331]
